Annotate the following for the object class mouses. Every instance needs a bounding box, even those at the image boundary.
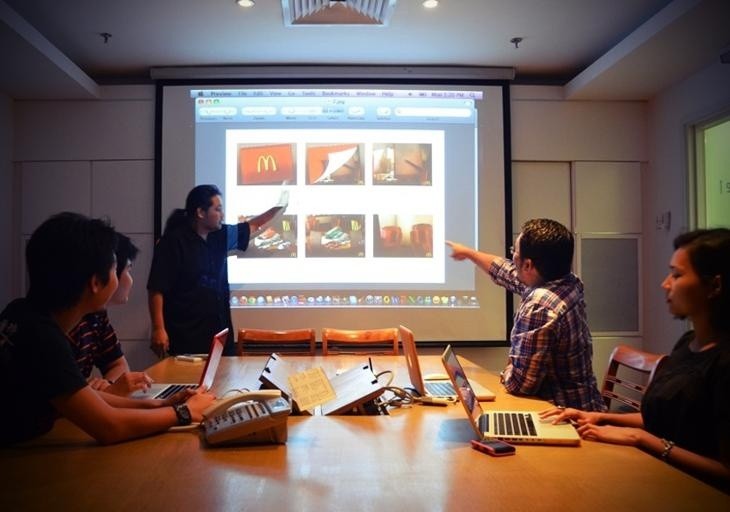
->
[424,373,450,380]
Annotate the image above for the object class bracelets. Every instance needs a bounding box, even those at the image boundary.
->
[662,438,675,460]
[108,381,114,385]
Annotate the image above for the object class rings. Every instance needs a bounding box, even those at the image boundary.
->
[557,405,565,410]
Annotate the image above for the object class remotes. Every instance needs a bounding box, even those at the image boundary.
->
[184,353,208,359]
[177,355,202,361]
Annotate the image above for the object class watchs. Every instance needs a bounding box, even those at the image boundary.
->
[173,403,192,426]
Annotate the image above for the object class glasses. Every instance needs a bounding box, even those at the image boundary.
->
[508,245,520,256]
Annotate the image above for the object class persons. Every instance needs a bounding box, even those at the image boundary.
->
[538,228,730,495]
[147,185,288,360]
[446,218,608,411]
[0,212,216,449]
[65,233,155,397]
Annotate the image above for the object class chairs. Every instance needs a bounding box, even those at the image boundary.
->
[237,327,317,356]
[599,343,668,412]
[321,327,399,356]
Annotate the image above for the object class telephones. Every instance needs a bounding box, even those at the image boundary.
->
[202,389,292,446]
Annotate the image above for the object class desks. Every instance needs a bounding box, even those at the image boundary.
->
[0,354,730,511]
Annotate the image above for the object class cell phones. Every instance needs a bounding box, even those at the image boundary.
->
[472,439,515,455]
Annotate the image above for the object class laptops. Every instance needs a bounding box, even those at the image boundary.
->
[129,328,229,400]
[441,344,581,446]
[399,325,495,401]
